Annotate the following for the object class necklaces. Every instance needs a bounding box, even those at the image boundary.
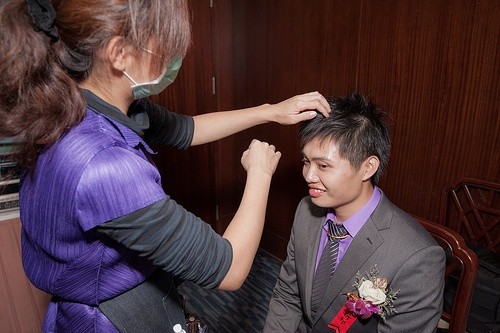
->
[327,231,351,239]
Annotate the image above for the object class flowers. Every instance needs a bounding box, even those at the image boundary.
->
[342,264,400,323]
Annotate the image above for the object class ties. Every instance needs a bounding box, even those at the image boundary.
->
[311,220,348,313]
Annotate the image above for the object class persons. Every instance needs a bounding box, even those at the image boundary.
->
[0,0,331,333]
[263,90,444,333]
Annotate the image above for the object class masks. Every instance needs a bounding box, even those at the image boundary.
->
[123,42,183,99]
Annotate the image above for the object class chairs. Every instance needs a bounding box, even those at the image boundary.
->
[408,213,479,333]
[438,178,500,255]
